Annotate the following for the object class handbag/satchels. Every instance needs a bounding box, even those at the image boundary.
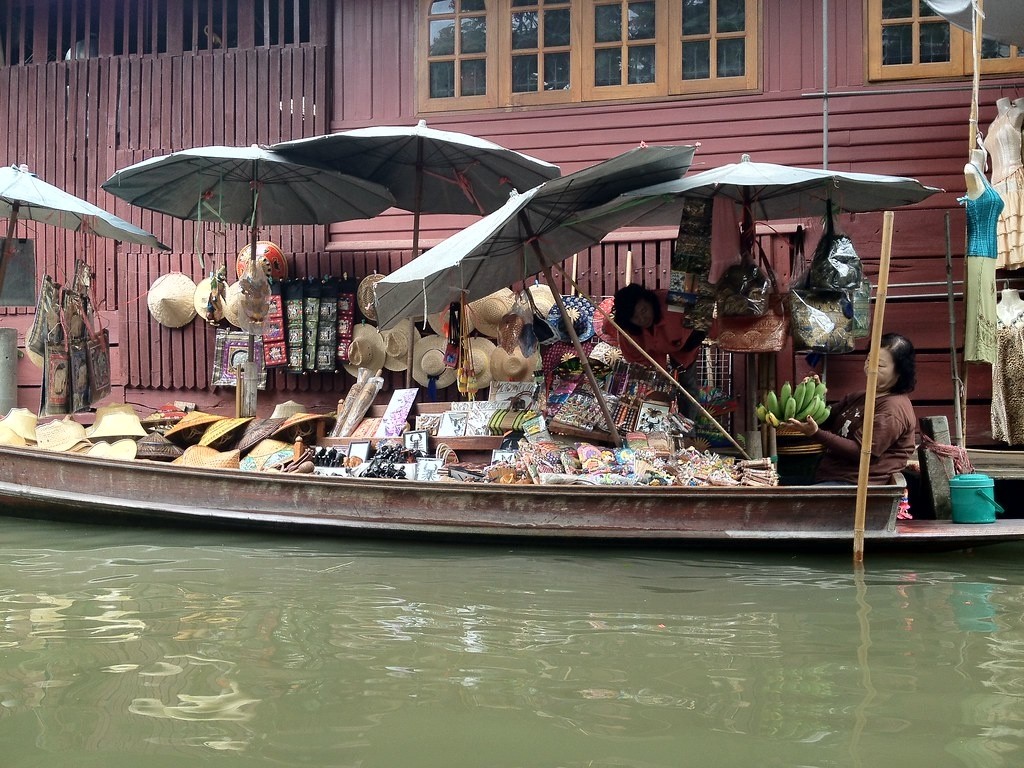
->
[846,274,871,339]
[28,275,110,415]
[790,287,856,354]
[488,391,537,436]
[810,231,862,289]
[715,263,768,316]
[719,238,787,353]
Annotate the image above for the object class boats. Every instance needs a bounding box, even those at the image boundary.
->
[0,400,908,554]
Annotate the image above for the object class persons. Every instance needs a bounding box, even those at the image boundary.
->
[988,288,1024,448]
[958,147,1005,365]
[775,331,917,487]
[984,96,1024,271]
[602,283,710,448]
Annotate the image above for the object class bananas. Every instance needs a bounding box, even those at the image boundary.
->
[756,377,831,428]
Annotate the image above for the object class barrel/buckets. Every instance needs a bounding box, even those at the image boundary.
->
[949,474,1004,523]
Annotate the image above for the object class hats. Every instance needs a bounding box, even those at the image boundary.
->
[342,274,619,393]
[0,401,337,469]
[147,241,287,327]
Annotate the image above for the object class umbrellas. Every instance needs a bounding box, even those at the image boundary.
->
[370,139,701,448]
[0,164,172,296]
[562,155,947,430]
[331,368,383,439]
[99,144,395,364]
[259,118,562,388]
